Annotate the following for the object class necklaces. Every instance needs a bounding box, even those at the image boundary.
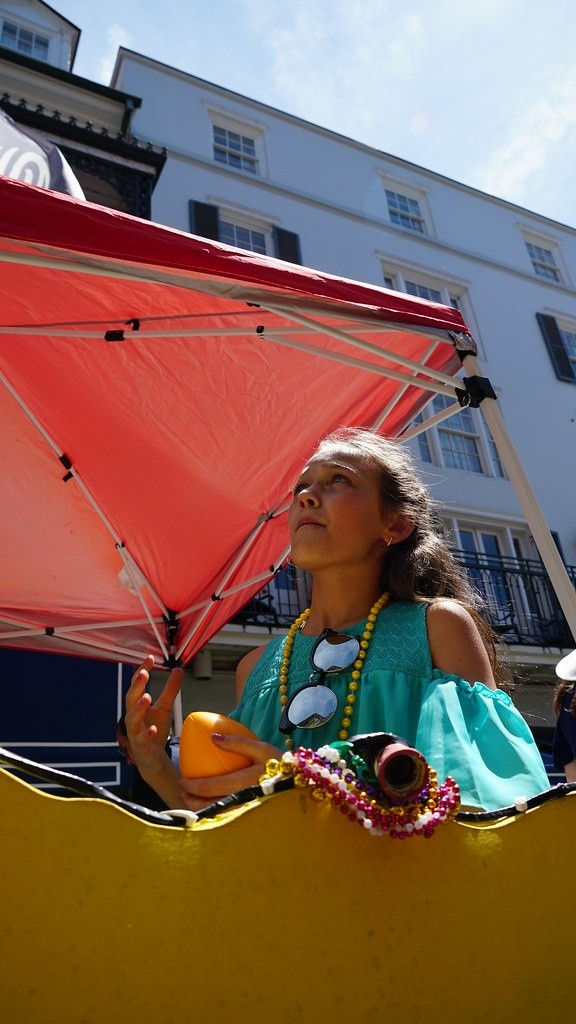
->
[279,593,389,758]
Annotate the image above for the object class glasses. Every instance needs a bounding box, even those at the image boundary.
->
[278,628,360,735]
[117,747,127,756]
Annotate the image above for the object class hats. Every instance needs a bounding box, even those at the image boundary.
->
[556,648,576,680]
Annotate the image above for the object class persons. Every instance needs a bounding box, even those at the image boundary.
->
[125,427,553,821]
[115,710,186,832]
[550,653,576,791]
